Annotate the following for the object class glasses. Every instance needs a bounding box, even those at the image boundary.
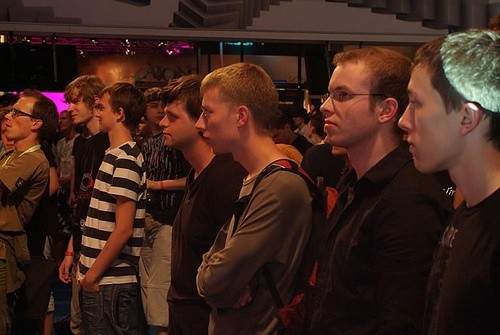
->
[321,89,389,102]
[146,103,166,109]
[10,108,41,119]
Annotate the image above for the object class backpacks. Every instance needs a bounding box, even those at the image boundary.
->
[232,158,338,335]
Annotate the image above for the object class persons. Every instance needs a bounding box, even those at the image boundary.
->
[397,29,500,335]
[195,62,314,335]
[0,89,59,335]
[59,74,104,335]
[75,81,146,335]
[0,91,356,335]
[307,47,453,335]
[159,73,248,335]
